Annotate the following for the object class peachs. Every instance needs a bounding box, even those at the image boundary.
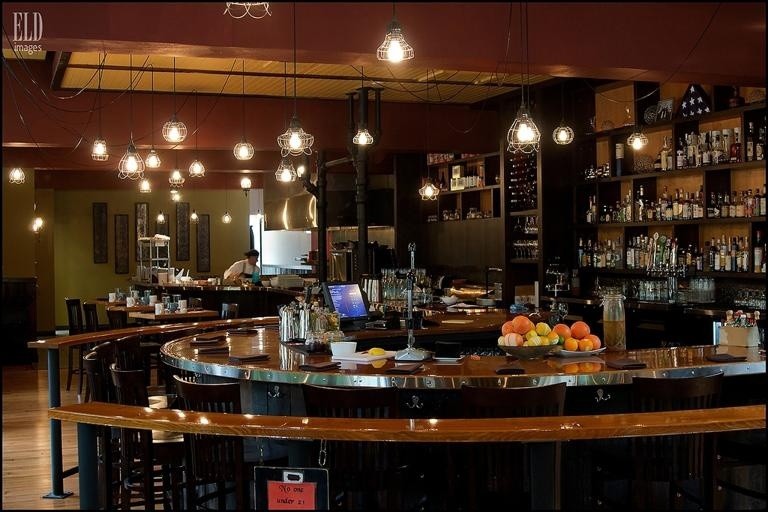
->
[553,321,571,339]
[570,321,591,339]
[584,335,602,350]
[501,316,531,347]
[592,363,602,372]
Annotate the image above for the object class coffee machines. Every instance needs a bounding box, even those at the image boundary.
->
[546,252,580,296]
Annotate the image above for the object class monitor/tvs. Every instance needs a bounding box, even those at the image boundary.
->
[322,279,370,331]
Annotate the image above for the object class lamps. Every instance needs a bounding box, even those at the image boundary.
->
[1,1,650,234]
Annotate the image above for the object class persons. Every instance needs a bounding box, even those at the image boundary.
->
[220,250,261,284]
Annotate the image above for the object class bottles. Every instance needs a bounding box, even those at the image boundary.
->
[603,293,628,351]
[279,305,344,352]
[135,265,175,284]
[727,308,764,348]
[508,155,538,206]
[577,121,767,272]
[426,154,493,221]
[570,268,581,297]
[378,267,433,302]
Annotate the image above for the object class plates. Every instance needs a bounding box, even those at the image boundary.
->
[432,355,465,363]
[558,347,605,357]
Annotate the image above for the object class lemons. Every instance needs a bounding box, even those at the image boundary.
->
[368,347,385,356]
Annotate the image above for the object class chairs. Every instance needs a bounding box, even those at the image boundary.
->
[29,283,767,512]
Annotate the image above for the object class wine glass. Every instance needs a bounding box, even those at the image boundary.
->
[513,216,538,260]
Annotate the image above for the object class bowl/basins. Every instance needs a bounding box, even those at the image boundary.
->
[440,297,459,306]
[494,345,557,361]
[330,340,357,357]
[261,281,271,287]
[332,360,357,371]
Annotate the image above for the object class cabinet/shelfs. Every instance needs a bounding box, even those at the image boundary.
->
[568,77,765,304]
[315,150,427,279]
[493,93,596,304]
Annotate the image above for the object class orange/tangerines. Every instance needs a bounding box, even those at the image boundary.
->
[558,336,564,345]
[523,322,559,346]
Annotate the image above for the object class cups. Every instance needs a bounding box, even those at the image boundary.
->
[400,307,424,329]
[591,274,768,305]
[107,288,190,314]
